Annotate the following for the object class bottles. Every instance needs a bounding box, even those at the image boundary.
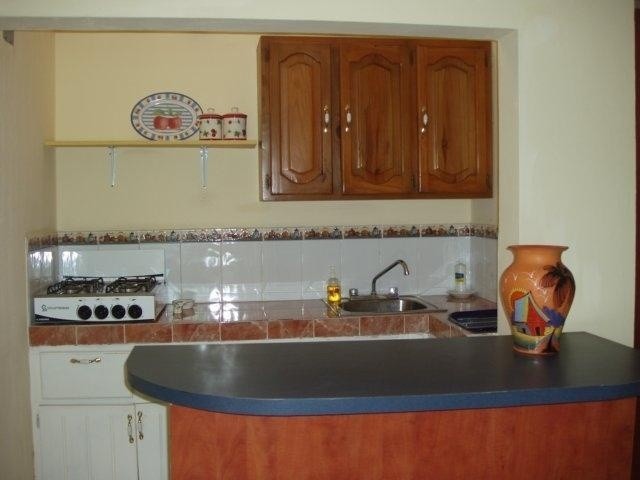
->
[326,265,341,303]
[197,106,247,140]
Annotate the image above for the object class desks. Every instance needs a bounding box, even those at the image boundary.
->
[125,331,640,480]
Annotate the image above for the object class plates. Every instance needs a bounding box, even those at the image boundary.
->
[131,92,203,141]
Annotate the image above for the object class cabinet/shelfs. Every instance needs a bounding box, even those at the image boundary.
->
[255,34,493,202]
[27,343,170,480]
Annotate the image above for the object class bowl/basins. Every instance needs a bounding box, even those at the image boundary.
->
[449,290,476,300]
[172,299,194,309]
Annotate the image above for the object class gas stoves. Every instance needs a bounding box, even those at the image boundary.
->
[33,276,167,323]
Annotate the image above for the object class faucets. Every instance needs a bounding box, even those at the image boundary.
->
[370,257,410,295]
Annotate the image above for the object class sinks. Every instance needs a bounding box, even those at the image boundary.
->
[338,295,448,316]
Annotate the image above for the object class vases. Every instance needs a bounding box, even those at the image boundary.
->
[498,244,577,355]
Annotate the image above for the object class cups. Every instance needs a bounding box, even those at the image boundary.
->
[173,301,184,314]
